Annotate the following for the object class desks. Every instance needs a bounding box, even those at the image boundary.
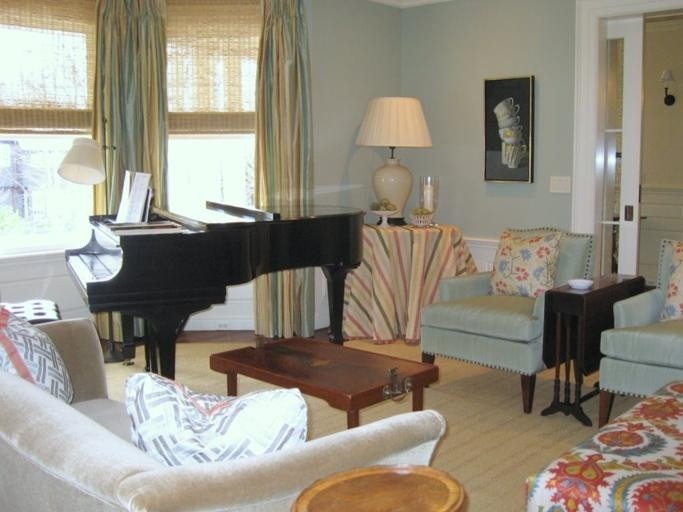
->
[335,224,481,347]
[538,271,646,429]
[522,377,683,512]
[207,335,441,430]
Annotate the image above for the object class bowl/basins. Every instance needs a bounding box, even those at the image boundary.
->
[565,278,594,290]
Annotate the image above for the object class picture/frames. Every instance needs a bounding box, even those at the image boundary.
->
[482,73,537,185]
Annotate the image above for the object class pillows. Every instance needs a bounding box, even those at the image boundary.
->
[487,228,563,300]
[657,238,682,323]
[0,303,77,405]
[123,369,310,470]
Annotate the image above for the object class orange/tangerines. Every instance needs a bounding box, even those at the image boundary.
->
[370,199,397,211]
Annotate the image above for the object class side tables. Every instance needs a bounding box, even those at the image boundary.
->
[292,464,469,512]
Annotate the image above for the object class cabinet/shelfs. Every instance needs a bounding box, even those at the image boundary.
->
[637,183,682,285]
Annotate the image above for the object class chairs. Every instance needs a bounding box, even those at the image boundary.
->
[418,228,597,415]
[592,238,683,429]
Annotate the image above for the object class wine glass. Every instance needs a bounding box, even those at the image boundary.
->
[370,210,399,227]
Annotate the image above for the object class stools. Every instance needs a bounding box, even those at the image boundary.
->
[3,298,64,325]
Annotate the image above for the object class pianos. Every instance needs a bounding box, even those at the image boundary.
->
[66,200,366,380]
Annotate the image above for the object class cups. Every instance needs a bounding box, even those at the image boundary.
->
[419,175,437,215]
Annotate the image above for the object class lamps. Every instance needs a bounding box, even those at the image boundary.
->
[353,93,436,219]
[656,66,677,107]
[55,136,126,366]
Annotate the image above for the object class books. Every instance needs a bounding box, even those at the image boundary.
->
[112,169,153,224]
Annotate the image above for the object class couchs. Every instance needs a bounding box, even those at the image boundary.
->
[0,314,450,512]
